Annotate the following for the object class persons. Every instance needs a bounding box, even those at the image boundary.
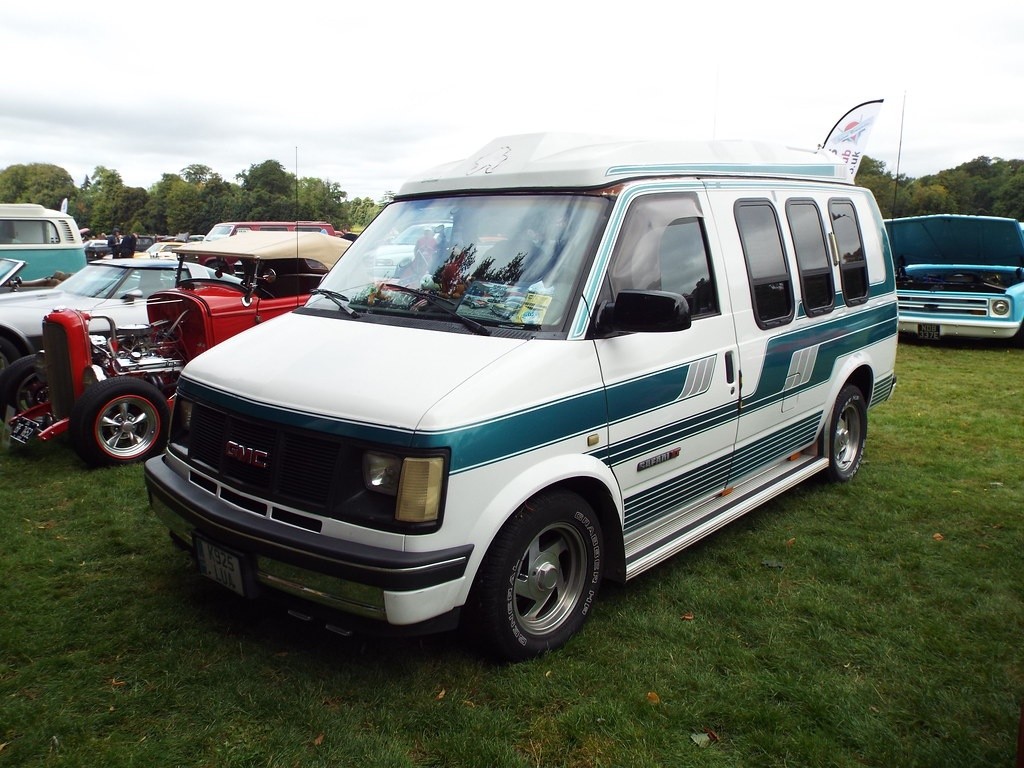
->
[107,227,137,259]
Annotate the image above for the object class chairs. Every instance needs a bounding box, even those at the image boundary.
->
[46,272,72,286]
[659,217,715,315]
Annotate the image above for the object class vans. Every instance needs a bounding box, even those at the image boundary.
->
[0,202,88,282]
[143,131,899,666]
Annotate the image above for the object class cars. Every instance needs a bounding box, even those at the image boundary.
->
[0,221,452,467]
[882,212,1024,350]
